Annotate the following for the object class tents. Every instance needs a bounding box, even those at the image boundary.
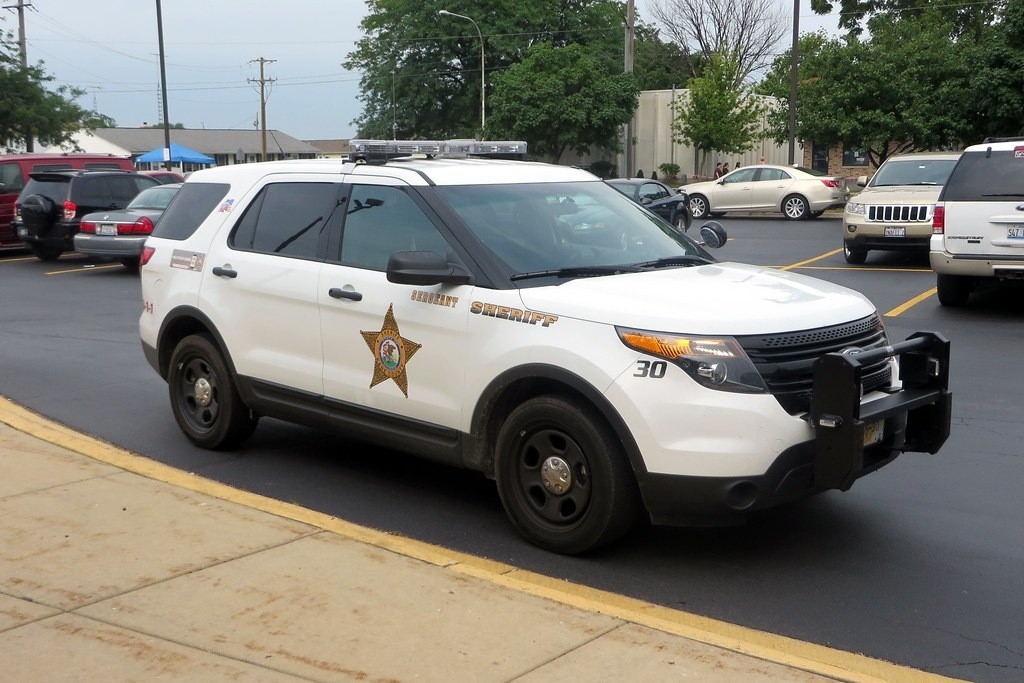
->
[133,143,216,173]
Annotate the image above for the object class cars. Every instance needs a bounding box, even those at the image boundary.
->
[679,164,851,220]
[138,170,184,185]
[74,182,184,271]
[550,177,693,235]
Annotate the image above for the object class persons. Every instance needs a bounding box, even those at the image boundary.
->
[735,162,740,170]
[722,162,729,175]
[713,162,722,180]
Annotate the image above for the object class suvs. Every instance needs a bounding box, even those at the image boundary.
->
[843,152,967,265]
[0,150,139,255]
[929,136,1024,306]
[11,171,164,262]
[138,159,955,557]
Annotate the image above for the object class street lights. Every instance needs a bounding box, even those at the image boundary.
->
[439,10,484,133]
